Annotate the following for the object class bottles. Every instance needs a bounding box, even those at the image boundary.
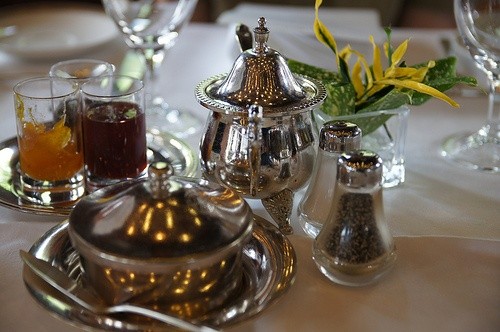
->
[312,151,399,288]
[296,120,362,238]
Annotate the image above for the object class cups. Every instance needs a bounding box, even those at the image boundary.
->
[81,75,149,193]
[12,76,86,205]
[49,58,116,115]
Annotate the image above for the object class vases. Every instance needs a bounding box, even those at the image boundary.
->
[312,108,409,189]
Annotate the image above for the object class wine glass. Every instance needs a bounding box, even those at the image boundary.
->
[439,1,500,173]
[102,0,205,140]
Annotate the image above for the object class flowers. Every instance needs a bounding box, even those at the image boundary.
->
[284,0,487,137]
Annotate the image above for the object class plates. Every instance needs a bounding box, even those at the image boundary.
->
[0,126,196,215]
[0,8,120,62]
[21,214,297,332]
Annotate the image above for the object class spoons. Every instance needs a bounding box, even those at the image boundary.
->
[20,249,226,332]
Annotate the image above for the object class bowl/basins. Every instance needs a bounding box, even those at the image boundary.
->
[67,161,256,322]
